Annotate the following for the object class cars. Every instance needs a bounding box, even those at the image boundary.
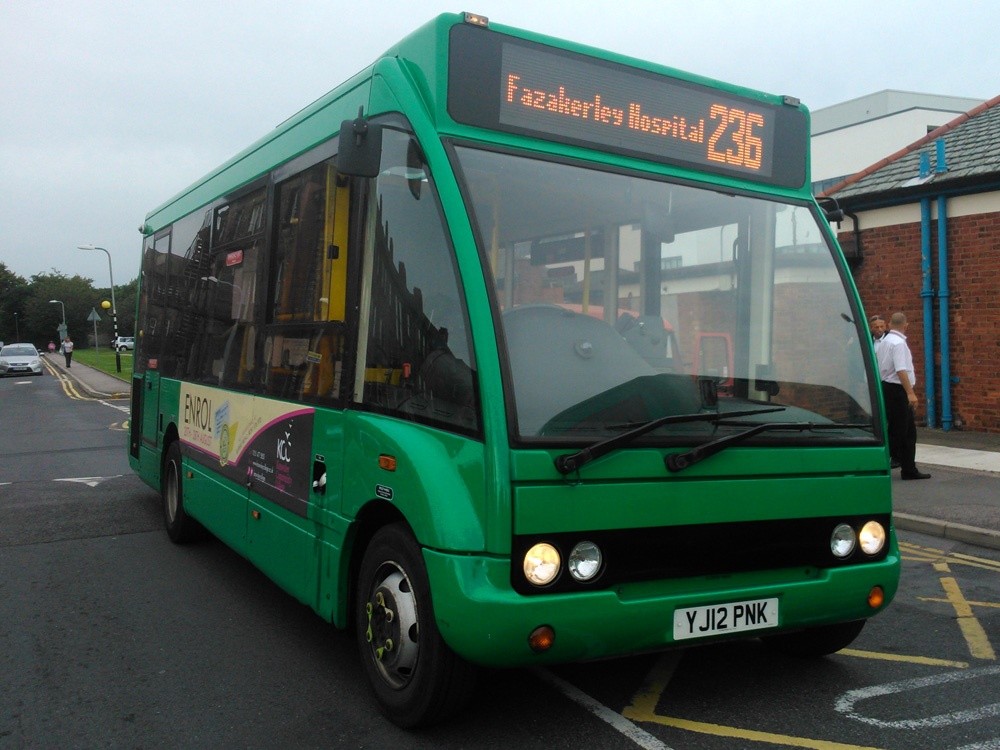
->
[0,343,45,377]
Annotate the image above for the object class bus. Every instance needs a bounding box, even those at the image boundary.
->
[126,15,903,722]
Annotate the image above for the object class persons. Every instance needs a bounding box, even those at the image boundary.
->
[419,250,528,409]
[63,337,74,368]
[845,312,931,480]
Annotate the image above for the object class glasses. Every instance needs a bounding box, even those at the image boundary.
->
[869,316,885,323]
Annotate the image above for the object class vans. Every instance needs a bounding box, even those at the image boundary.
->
[558,303,734,397]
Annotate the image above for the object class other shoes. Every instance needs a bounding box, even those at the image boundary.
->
[902,471,931,480]
[891,462,902,468]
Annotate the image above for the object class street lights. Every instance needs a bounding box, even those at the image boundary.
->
[14,312,18,341]
[202,277,244,387]
[77,245,122,373]
[49,300,65,340]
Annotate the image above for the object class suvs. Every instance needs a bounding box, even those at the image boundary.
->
[112,337,134,351]
